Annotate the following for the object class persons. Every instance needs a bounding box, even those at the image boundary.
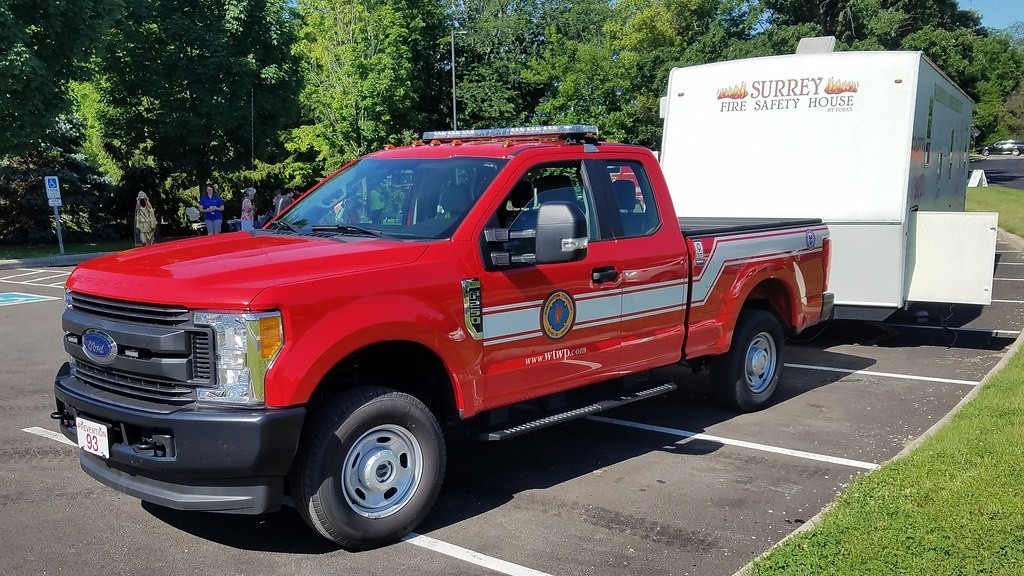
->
[331,189,346,224]
[184,203,204,238]
[272,185,306,214]
[135,191,156,246]
[199,185,224,235]
[368,182,386,225]
[241,188,256,230]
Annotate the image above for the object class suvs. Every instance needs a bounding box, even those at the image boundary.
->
[977,138,1024,156]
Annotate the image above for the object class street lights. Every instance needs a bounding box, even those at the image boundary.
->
[451,31,468,130]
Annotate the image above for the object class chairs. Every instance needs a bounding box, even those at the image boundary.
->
[505,176,583,252]
[611,180,648,236]
[406,183,474,237]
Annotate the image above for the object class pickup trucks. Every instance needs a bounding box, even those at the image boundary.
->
[52,126,836,548]
[607,147,660,197]
